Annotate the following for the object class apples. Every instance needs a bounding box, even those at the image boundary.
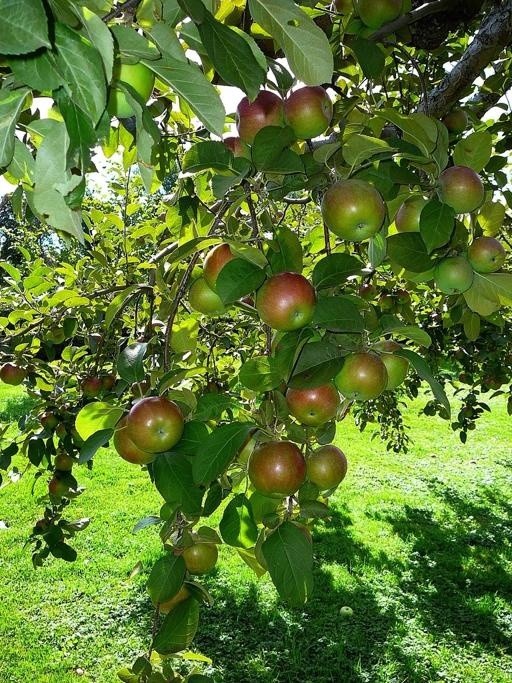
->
[340,606,354,618]
[149,539,218,613]
[1,58,512,499]
